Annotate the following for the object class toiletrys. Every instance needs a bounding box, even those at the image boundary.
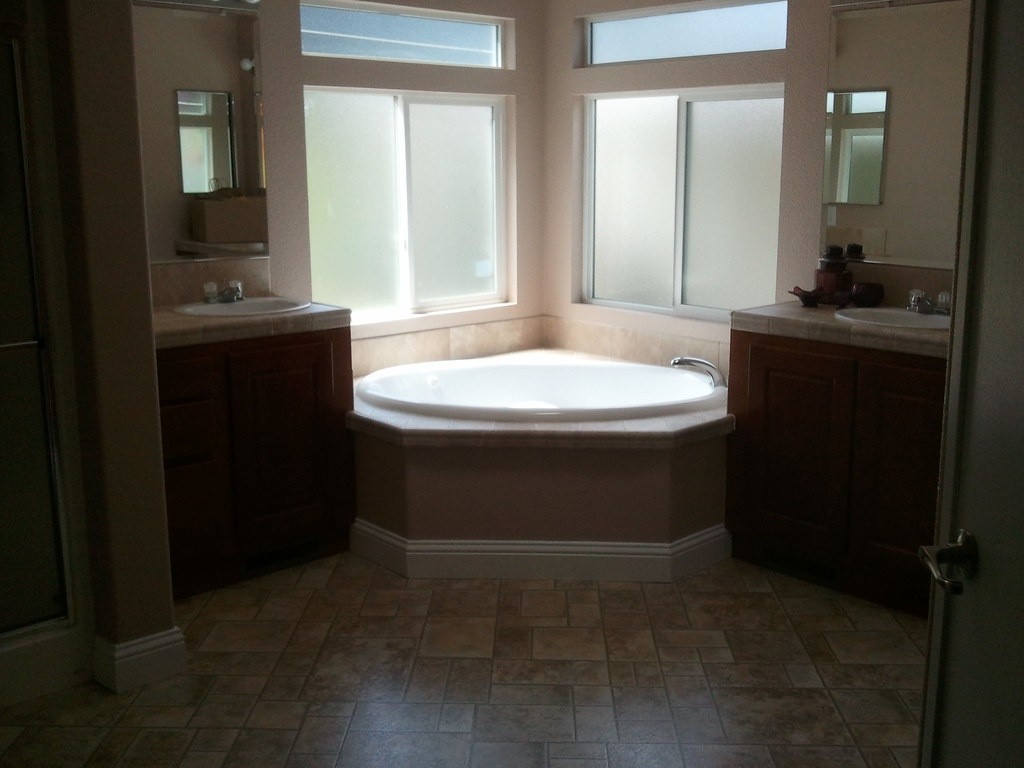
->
[845,243,865,259]
[817,244,846,269]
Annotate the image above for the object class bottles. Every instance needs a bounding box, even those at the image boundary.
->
[837,244,872,300]
[815,246,853,306]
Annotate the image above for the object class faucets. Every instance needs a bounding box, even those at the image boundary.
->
[670,354,727,388]
[906,289,950,317]
[204,279,245,304]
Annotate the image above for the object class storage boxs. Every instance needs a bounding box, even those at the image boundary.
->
[191,188,269,244]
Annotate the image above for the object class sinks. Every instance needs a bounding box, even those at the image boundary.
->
[833,306,950,330]
[171,297,312,317]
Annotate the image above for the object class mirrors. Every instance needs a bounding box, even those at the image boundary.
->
[820,89,889,207]
[176,90,234,196]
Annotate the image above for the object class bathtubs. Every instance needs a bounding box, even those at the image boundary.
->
[350,344,738,583]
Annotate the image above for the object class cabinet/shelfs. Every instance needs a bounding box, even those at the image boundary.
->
[156,326,356,603]
[724,330,947,619]
